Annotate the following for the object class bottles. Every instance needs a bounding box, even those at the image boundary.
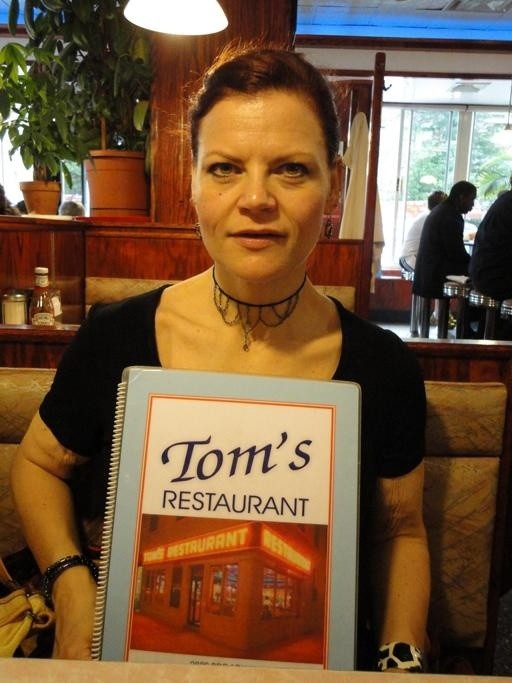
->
[1,265,62,327]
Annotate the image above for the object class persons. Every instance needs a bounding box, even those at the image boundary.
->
[0,184,85,217]
[398,177,512,341]
[9,47,432,677]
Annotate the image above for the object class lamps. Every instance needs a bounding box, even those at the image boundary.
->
[123,0,231,37]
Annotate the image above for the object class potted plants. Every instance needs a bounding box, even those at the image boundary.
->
[7,0,162,216]
[1,29,106,218]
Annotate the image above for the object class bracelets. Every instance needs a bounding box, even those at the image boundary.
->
[375,641,425,674]
[39,554,101,611]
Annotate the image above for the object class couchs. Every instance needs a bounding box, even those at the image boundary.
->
[82,270,360,371]
[1,337,510,683]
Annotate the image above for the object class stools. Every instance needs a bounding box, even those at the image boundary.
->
[398,266,512,342]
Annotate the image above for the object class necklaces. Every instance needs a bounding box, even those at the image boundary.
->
[211,264,309,354]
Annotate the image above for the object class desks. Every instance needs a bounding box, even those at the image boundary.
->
[1,319,84,369]
